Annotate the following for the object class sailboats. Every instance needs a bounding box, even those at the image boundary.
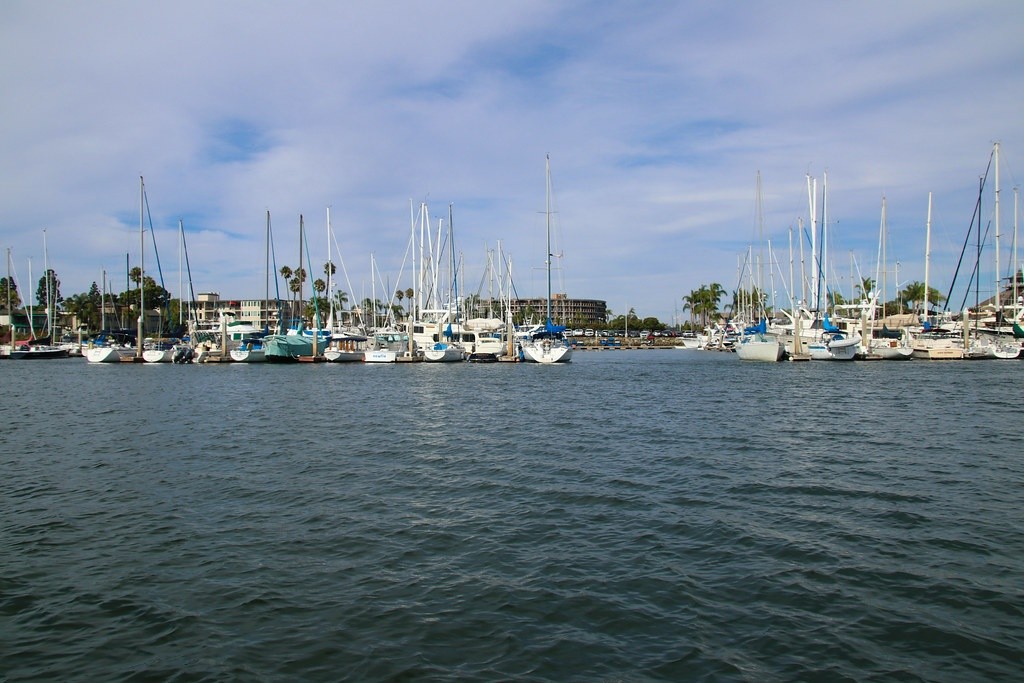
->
[1,155,578,363]
[679,139,1023,362]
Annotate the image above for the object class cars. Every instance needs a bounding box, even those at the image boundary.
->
[562,328,702,337]
